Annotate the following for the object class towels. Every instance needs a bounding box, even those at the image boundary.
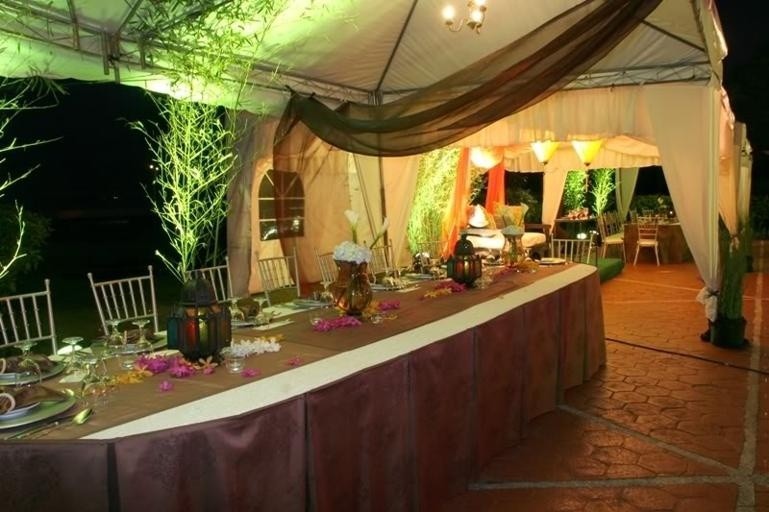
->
[124,328,160,345]
[238,297,261,316]
[1,351,66,412]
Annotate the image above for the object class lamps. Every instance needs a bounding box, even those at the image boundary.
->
[569,138,601,168]
[530,137,560,167]
[440,1,487,37]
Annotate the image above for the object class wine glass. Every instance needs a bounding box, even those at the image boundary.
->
[381,266,409,293]
[226,295,270,331]
[318,280,336,312]
[13,318,153,414]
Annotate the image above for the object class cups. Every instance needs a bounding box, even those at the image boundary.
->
[629,205,676,223]
[309,306,325,327]
[225,342,246,373]
[370,312,385,327]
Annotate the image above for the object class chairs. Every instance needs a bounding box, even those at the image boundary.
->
[85,265,159,341]
[253,247,304,307]
[360,238,403,281]
[181,256,245,323]
[0,279,59,359]
[467,204,690,266]
[415,233,450,273]
[313,244,343,297]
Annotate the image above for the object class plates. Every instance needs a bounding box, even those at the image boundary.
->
[405,273,447,279]
[292,299,331,307]
[0,358,75,430]
[117,330,167,354]
[535,257,567,264]
[482,257,502,265]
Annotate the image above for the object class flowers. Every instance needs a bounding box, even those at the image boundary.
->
[309,297,400,332]
[219,329,308,376]
[335,208,391,308]
[116,349,219,392]
[419,278,463,300]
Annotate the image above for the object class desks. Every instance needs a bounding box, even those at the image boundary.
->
[0,259,607,512]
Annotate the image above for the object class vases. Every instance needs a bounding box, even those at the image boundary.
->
[330,257,373,316]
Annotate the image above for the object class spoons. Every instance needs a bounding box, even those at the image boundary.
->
[29,408,92,440]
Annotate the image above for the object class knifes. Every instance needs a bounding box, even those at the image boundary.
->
[3,414,76,441]
